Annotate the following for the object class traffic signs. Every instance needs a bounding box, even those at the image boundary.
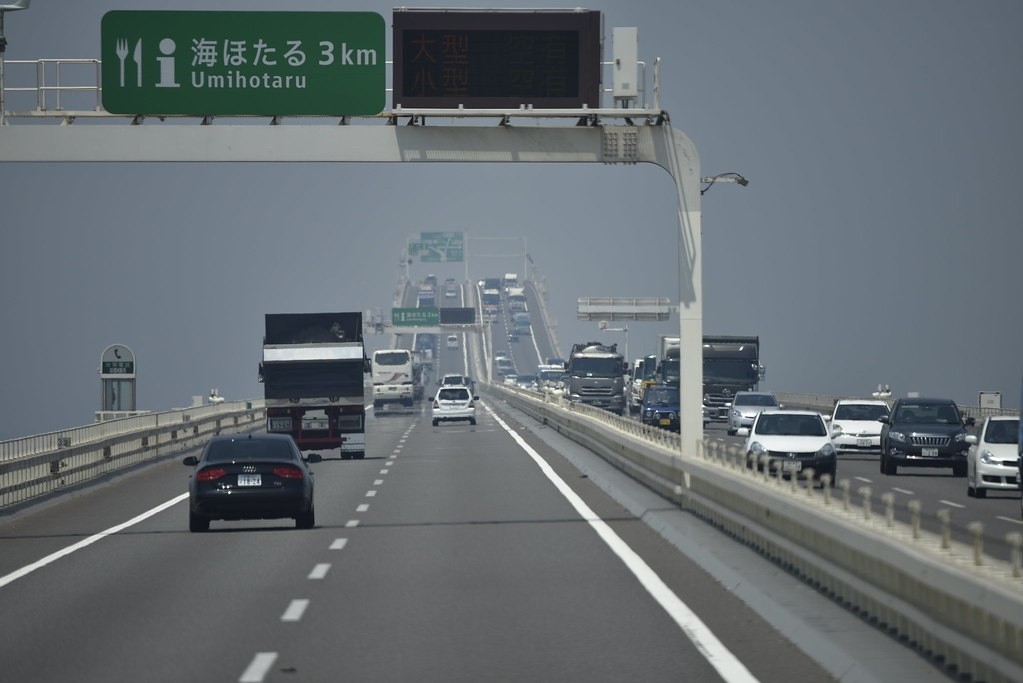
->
[410,231,465,262]
[101,11,387,117]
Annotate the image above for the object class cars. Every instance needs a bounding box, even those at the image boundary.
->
[824,400,891,454]
[495,351,569,396]
[428,384,479,426]
[727,391,786,436]
[965,416,1023,497]
[445,277,456,297]
[640,388,680,431]
[447,335,459,349]
[879,399,975,478]
[419,274,437,306]
[183,434,323,532]
[738,411,841,488]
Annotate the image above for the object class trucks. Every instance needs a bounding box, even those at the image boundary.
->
[257,312,370,460]
[372,334,436,407]
[478,273,501,323]
[563,335,766,429]
[505,273,533,342]
